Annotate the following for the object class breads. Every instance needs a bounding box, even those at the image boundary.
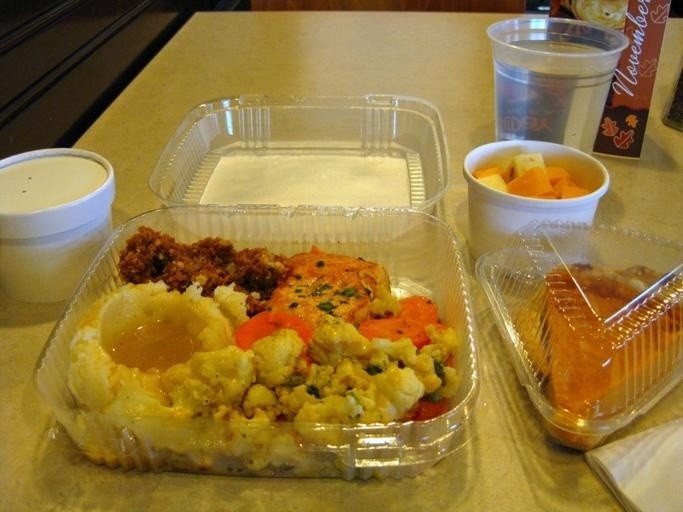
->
[509,261,683,450]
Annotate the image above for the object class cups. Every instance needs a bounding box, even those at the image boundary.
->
[462,139,609,263]
[0,146,115,308]
[483,14,632,146]
